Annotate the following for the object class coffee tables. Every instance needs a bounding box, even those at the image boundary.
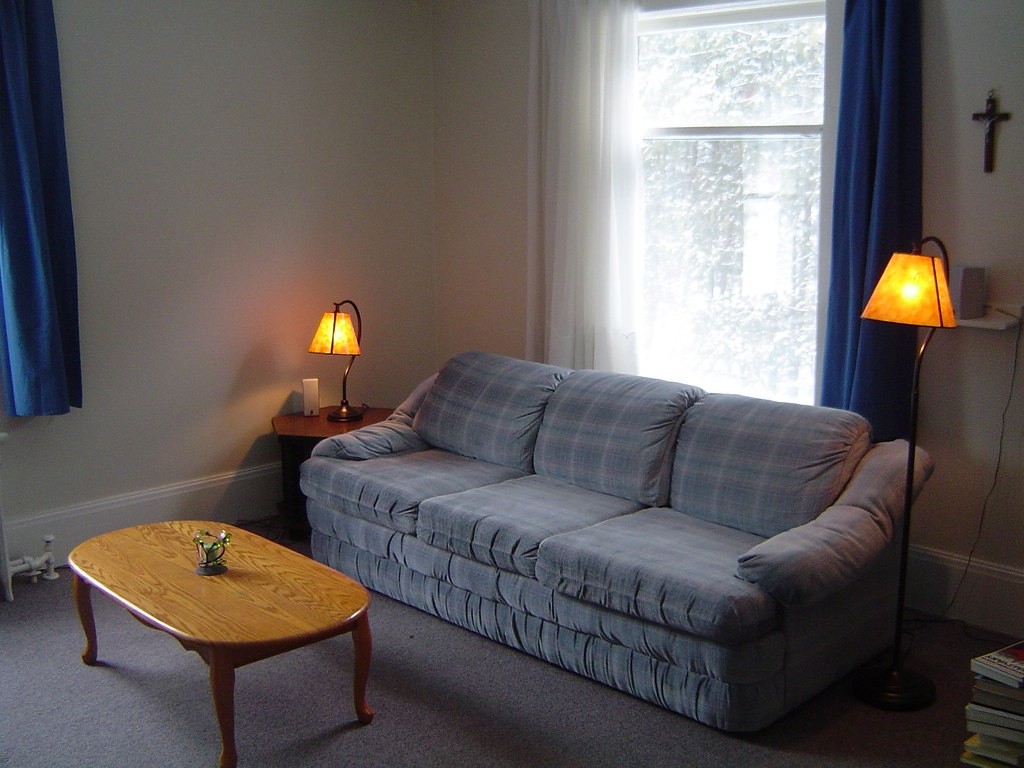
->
[67,520,375,768]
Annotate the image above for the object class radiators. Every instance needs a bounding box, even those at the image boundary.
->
[0,509,14,602]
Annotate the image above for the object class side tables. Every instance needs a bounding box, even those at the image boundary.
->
[273,407,396,541]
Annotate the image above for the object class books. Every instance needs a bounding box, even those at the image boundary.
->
[960,640,1024,768]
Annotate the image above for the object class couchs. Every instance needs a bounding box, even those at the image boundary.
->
[300,350,938,734]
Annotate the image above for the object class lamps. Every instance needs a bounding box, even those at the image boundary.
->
[308,300,363,423]
[861,237,958,711]
